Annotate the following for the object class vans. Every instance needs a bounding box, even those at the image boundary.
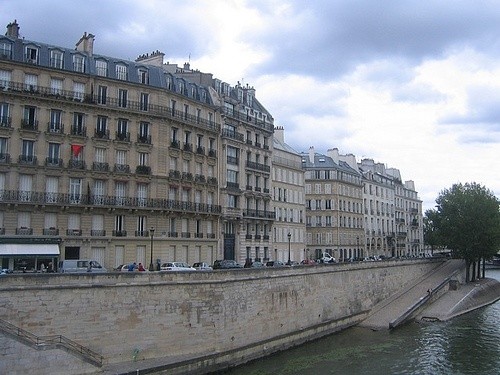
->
[57,260,107,273]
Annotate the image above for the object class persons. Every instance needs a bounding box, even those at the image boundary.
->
[41,262,52,273]
[87,262,92,272]
[128,262,146,271]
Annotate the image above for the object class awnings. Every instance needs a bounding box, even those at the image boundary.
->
[0,244,60,254]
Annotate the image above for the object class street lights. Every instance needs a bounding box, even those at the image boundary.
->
[356,235,360,262]
[286,229,292,265]
[148,225,155,272]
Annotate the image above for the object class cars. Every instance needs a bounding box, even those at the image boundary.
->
[192,251,432,270]
[113,264,148,272]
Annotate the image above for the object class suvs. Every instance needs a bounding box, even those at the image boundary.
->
[161,261,196,271]
